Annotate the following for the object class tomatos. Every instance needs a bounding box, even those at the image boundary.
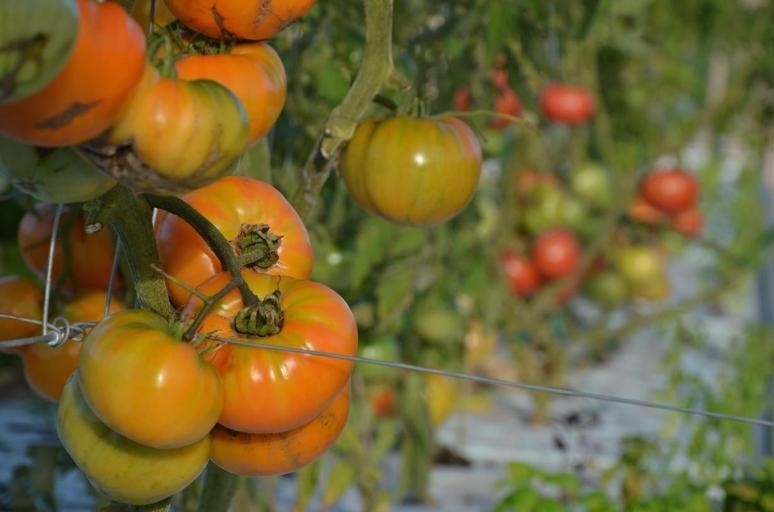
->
[1,0,707,507]
